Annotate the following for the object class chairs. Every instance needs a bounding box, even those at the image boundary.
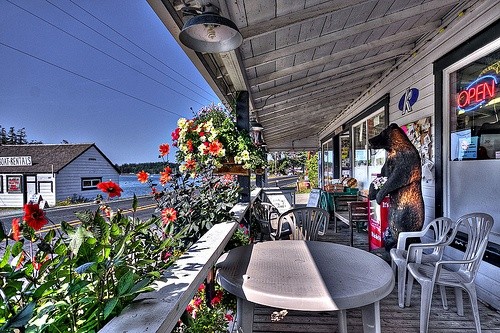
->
[390,217,453,310]
[253,202,329,241]
[405,213,494,333]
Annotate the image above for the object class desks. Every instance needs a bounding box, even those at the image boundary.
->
[216,240,395,333]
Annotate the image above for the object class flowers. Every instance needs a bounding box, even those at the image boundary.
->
[0,102,267,333]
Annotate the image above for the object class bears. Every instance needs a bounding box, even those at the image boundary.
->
[368,123,425,254]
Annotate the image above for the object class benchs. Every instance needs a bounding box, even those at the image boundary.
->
[333,190,369,247]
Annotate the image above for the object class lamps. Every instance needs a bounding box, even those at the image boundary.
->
[249,117,269,154]
[179,3,243,54]
